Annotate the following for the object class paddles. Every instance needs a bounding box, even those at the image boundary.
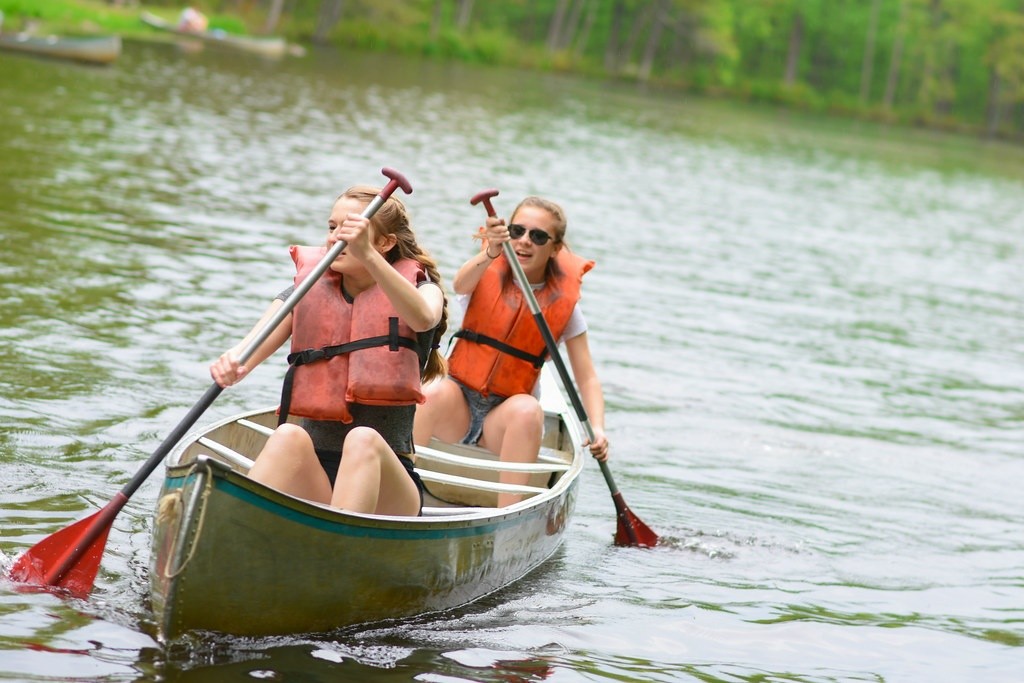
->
[469,188,656,547]
[7,166,414,598]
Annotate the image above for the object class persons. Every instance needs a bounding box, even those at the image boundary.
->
[209,186,449,516]
[411,196,609,509]
[177,0,209,34]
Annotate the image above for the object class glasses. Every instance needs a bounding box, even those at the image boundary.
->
[508,223,554,246]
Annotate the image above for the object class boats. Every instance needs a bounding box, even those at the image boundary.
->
[150,363,586,640]
[139,10,286,61]
[0,29,122,64]
